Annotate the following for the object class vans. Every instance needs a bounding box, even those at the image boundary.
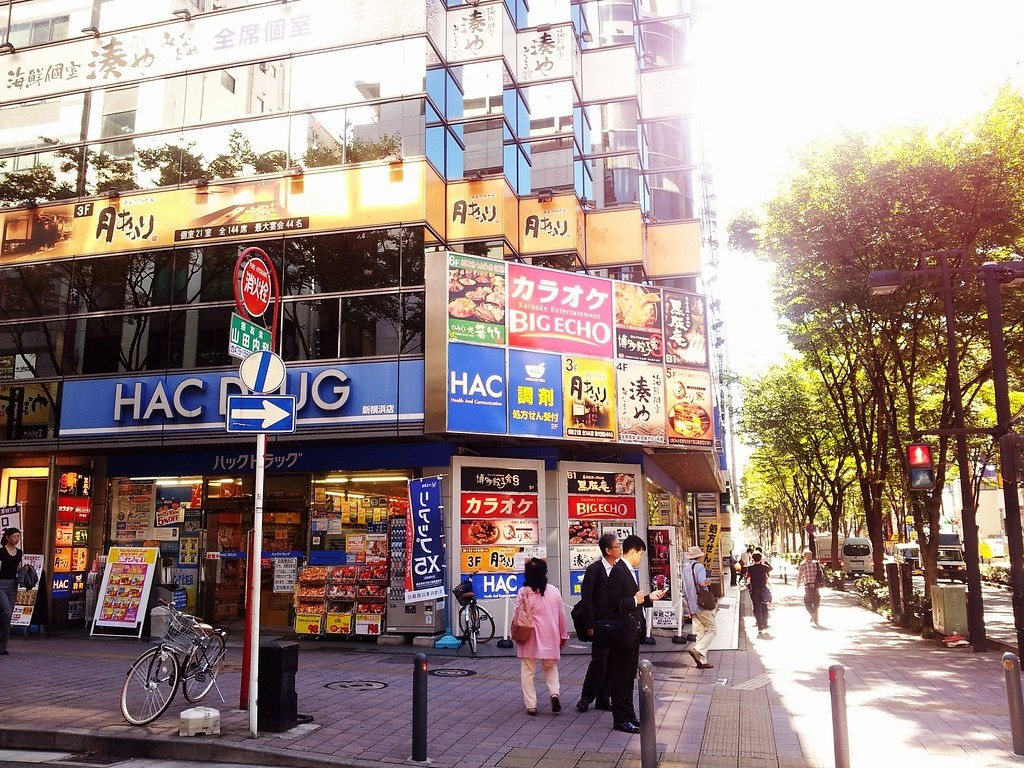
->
[841,538,874,578]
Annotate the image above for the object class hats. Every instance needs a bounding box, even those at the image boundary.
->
[687,546,705,560]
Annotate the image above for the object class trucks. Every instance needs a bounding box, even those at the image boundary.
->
[918,533,966,584]
[814,535,845,569]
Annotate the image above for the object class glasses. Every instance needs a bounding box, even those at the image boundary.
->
[612,544,621,548]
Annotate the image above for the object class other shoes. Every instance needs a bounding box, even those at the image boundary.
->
[595,700,613,712]
[614,721,640,734]
[759,627,764,630]
[763,625,769,629]
[689,647,703,667]
[631,718,641,726]
[698,662,714,668]
[576,699,589,711]
[1,651,10,655]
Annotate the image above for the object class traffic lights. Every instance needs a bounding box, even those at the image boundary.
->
[1000,432,1022,483]
[906,443,936,490]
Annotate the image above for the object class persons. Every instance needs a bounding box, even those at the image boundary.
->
[608,535,666,734]
[797,549,825,625]
[510,557,570,715]
[748,553,773,630]
[729,546,767,589]
[682,546,718,668]
[0,528,38,655]
[576,533,622,712]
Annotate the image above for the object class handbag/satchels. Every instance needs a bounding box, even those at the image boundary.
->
[571,599,594,643]
[815,562,825,588]
[692,562,716,610]
[761,587,772,603]
[739,560,745,566]
[512,586,534,645]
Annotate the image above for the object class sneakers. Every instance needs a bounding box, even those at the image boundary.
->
[551,695,561,713]
[528,708,536,716]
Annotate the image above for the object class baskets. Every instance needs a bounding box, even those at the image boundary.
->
[453,581,473,606]
[160,610,210,655]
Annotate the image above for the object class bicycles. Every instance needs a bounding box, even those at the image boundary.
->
[450,570,496,655]
[120,597,231,726]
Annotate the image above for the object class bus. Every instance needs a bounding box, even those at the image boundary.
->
[893,544,923,575]
